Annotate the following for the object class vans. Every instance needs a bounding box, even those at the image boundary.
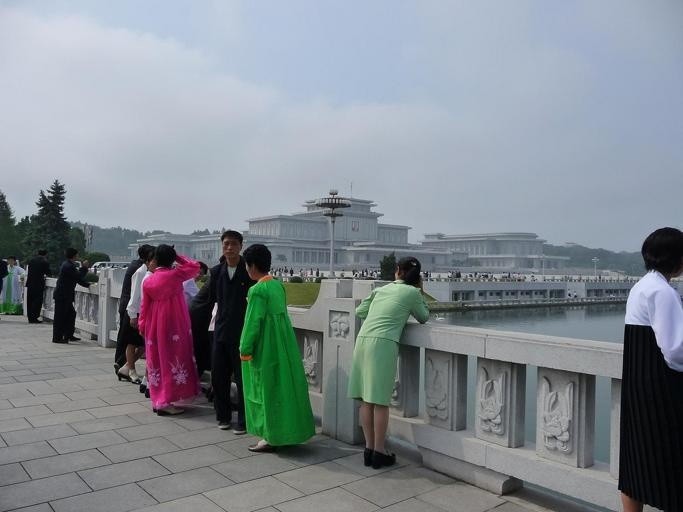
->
[90,261,132,272]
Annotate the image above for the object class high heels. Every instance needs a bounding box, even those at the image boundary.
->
[364,448,395,469]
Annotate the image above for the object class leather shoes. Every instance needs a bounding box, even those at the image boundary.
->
[248,439,269,451]
[114,362,150,398]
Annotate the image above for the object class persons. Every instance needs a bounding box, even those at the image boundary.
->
[0,243,215,417]
[209,230,257,435]
[346,256,429,469]
[616,226,683,512]
[238,243,317,452]
[269,261,682,285]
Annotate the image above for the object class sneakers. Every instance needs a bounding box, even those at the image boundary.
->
[53,336,81,343]
[233,426,246,434]
[153,404,184,416]
[217,421,230,428]
[29,318,42,323]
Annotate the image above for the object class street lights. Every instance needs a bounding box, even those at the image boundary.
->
[591,256,599,279]
[315,188,351,273]
